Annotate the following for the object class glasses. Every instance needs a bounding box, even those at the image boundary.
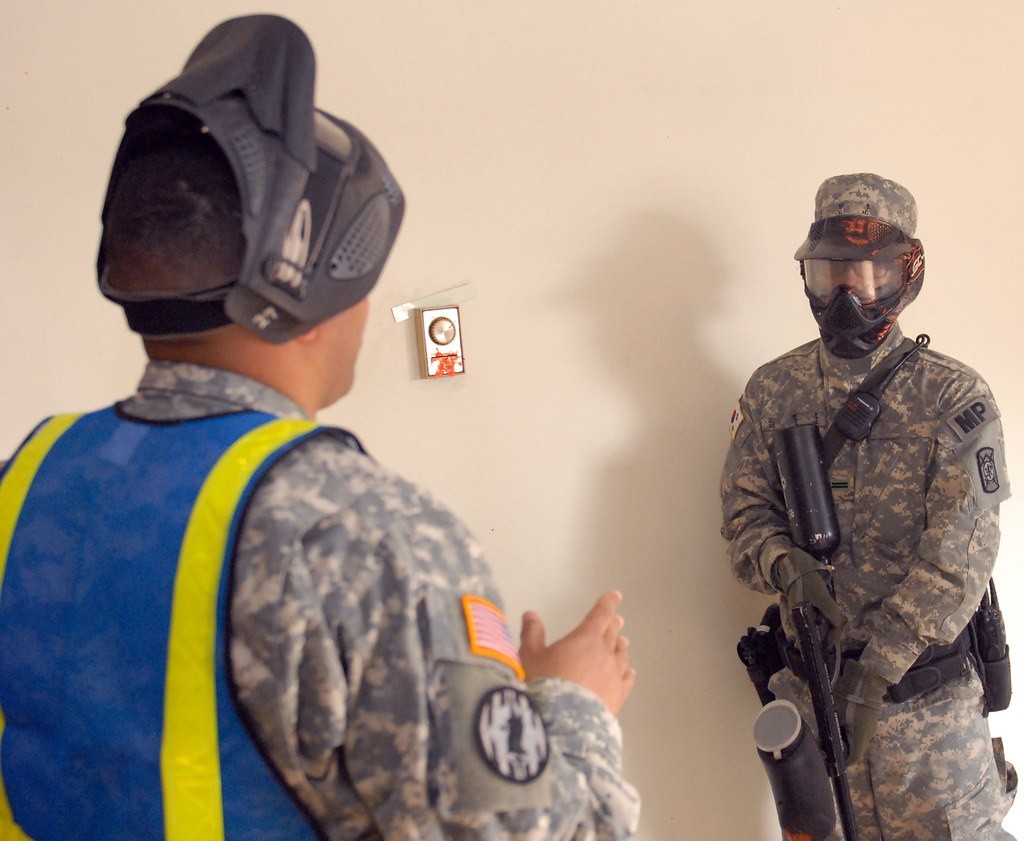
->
[814,259,894,279]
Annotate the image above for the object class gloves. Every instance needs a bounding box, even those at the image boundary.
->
[779,548,842,641]
[820,659,887,767]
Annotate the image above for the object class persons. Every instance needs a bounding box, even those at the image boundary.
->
[0,96,641,841]
[720,173,1018,841]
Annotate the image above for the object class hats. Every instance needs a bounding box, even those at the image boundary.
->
[815,173,917,240]
